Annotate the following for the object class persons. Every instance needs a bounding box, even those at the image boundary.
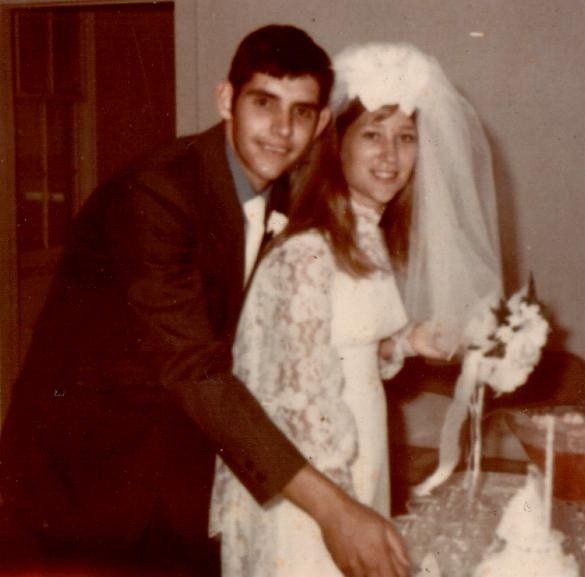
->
[0,23,336,577]
[207,40,505,577]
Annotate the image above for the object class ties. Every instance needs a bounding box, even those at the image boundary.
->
[244,195,265,288]
